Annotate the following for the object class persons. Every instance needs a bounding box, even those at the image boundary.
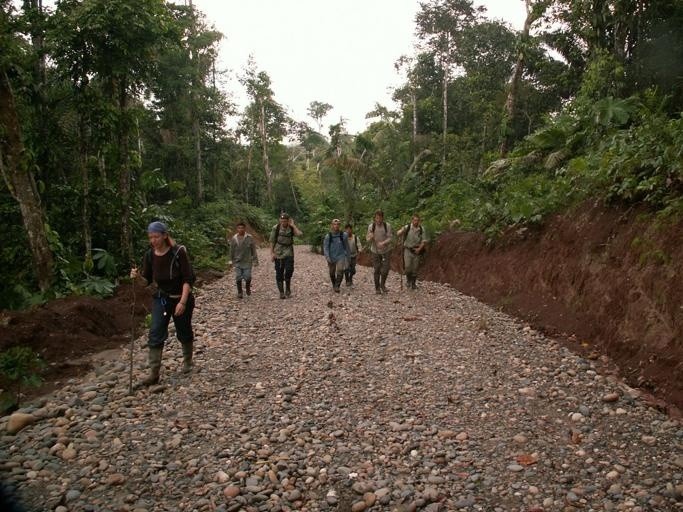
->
[341,223,362,287]
[229,223,258,299]
[323,219,351,293]
[365,208,393,294]
[130,220,195,386]
[269,212,303,299]
[396,214,427,290]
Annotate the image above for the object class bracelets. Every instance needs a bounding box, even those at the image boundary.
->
[178,301,187,307]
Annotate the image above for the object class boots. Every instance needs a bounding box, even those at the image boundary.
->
[380,273,389,292]
[331,272,354,292]
[278,280,291,298]
[405,274,419,289]
[182,342,193,373]
[245,280,251,296]
[138,349,162,385]
[374,273,381,294]
[236,280,243,298]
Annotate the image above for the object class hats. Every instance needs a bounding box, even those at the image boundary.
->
[147,222,167,233]
[281,213,290,219]
[333,219,341,224]
